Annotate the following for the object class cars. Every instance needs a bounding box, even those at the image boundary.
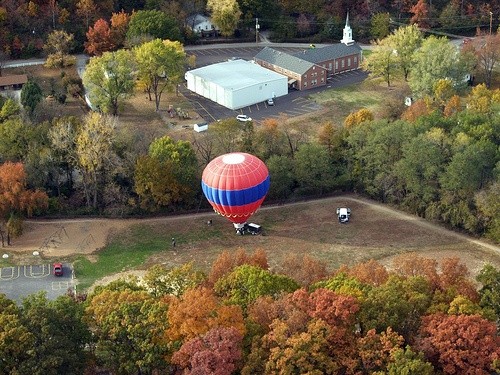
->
[267,99,274,105]
[236,115,253,123]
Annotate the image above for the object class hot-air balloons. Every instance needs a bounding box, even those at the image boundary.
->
[201,152,271,235]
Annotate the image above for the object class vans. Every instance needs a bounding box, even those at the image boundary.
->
[336,207,350,223]
[53,263,63,276]
